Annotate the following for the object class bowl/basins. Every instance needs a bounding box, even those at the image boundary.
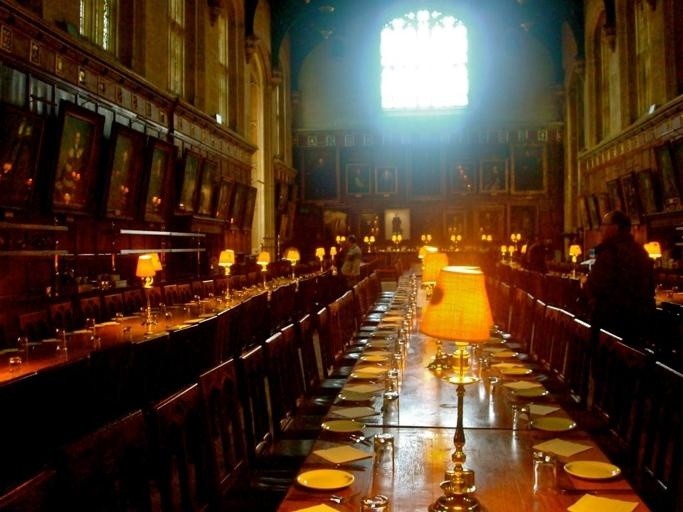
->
[654,288,683,305]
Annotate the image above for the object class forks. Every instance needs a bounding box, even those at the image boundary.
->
[320,434,375,443]
[287,489,363,505]
[329,434,375,441]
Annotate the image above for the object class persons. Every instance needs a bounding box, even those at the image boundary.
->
[339,233,360,286]
[581,210,656,344]
[526,233,548,272]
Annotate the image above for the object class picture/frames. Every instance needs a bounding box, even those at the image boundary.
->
[37,98,257,234]
[300,140,682,241]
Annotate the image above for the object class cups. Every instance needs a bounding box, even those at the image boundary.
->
[8,271,320,377]
[359,494,389,512]
[383,392,399,417]
[485,357,503,385]
[383,280,417,392]
[530,451,557,495]
[510,402,531,436]
[374,432,395,469]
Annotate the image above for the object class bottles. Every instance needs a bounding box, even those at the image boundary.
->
[0,230,63,250]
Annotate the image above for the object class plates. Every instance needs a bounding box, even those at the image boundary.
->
[511,388,549,398]
[350,286,404,380]
[320,420,364,433]
[528,416,577,433]
[498,367,533,376]
[562,460,623,482]
[338,391,375,403]
[482,326,511,346]
[490,352,519,359]
[294,467,354,492]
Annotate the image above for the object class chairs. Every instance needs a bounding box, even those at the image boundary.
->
[0,248,681,511]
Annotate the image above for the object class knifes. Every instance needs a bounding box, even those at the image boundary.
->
[299,462,368,471]
[559,487,635,496]
